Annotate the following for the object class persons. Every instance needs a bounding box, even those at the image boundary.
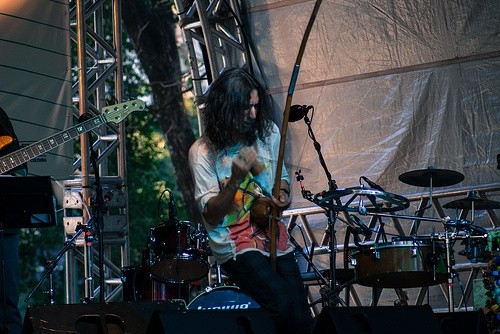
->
[0,107,28,334]
[188,67,313,334]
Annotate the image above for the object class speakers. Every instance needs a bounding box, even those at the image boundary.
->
[313,304,490,334]
[22,299,291,334]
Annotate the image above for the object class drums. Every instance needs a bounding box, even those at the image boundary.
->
[144,218,213,284]
[121,263,193,309]
[182,283,264,313]
[353,239,457,290]
[461,229,500,263]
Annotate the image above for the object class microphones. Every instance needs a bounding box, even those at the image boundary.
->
[77,113,93,124]
[288,105,314,122]
[288,223,297,233]
[353,216,372,236]
[361,176,384,192]
[169,192,177,217]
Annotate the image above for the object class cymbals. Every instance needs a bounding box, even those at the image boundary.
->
[397,168,465,188]
[441,197,500,211]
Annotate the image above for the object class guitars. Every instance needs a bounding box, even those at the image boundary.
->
[0,98,146,177]
